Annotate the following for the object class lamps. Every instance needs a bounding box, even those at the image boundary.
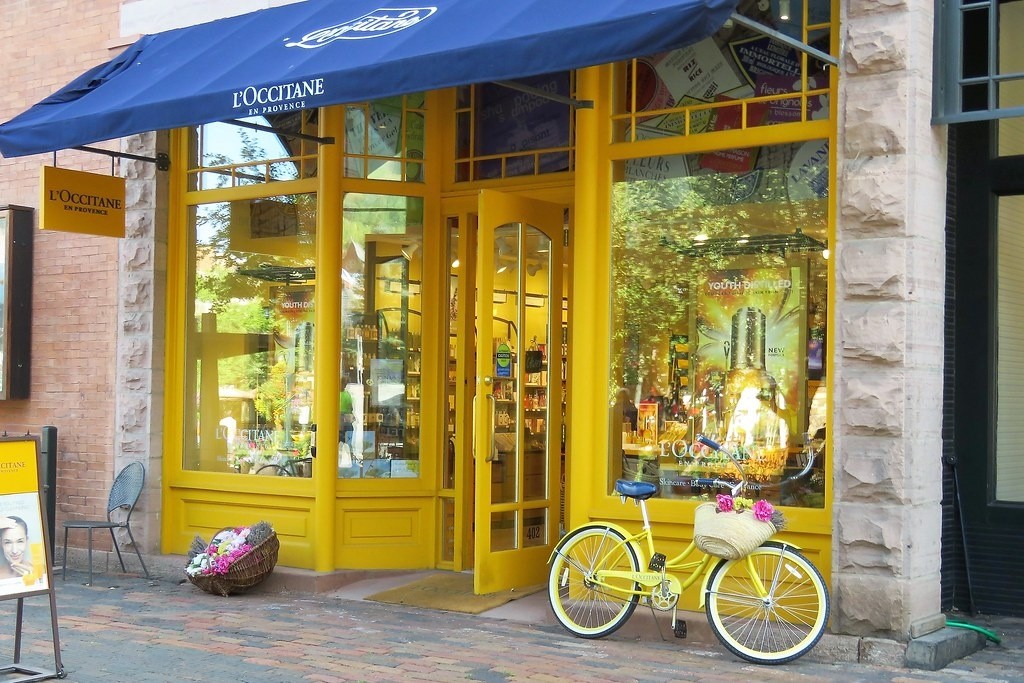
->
[721,0,791,28]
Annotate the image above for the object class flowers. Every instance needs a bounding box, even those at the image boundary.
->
[184,518,274,577]
[716,493,786,533]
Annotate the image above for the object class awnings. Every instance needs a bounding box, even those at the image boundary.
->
[0,0,839,165]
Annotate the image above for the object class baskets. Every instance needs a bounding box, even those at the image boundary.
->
[185,526,283,595]
[692,500,777,560]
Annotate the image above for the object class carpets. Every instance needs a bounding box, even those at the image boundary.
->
[362,573,549,615]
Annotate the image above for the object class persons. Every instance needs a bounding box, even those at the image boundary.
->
[0,515,33,580]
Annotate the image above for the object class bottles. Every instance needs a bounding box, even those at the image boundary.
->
[406,408,419,429]
[522,390,546,408]
[406,383,421,399]
[346,352,377,368]
[495,340,512,377]
[388,328,421,348]
[346,323,378,340]
[495,410,509,426]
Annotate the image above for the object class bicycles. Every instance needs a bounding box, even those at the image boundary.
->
[253,445,312,478]
[545,434,830,666]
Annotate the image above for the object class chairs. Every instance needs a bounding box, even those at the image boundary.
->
[62,460,150,588]
